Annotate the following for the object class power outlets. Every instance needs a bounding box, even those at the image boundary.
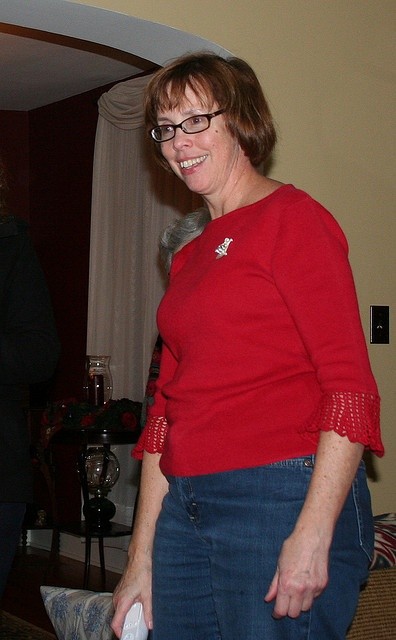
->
[370,306,389,344]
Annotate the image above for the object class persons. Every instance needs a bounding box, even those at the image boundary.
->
[108,49,384,640]
[0,163,61,611]
[138,207,210,432]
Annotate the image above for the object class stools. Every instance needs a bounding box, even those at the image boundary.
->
[42,425,141,582]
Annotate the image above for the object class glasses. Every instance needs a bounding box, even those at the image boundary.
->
[148,109,224,142]
